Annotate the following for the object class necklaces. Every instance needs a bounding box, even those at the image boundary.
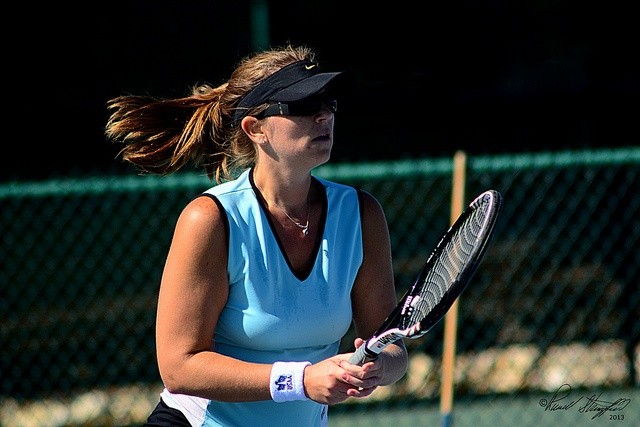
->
[251,167,314,237]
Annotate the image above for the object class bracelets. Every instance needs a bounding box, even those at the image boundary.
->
[268,360,311,404]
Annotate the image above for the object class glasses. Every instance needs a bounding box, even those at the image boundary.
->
[250,91,337,119]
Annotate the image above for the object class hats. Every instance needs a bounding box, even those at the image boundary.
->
[228,60,341,127]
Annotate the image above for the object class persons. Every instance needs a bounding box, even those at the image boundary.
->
[103,40,409,427]
